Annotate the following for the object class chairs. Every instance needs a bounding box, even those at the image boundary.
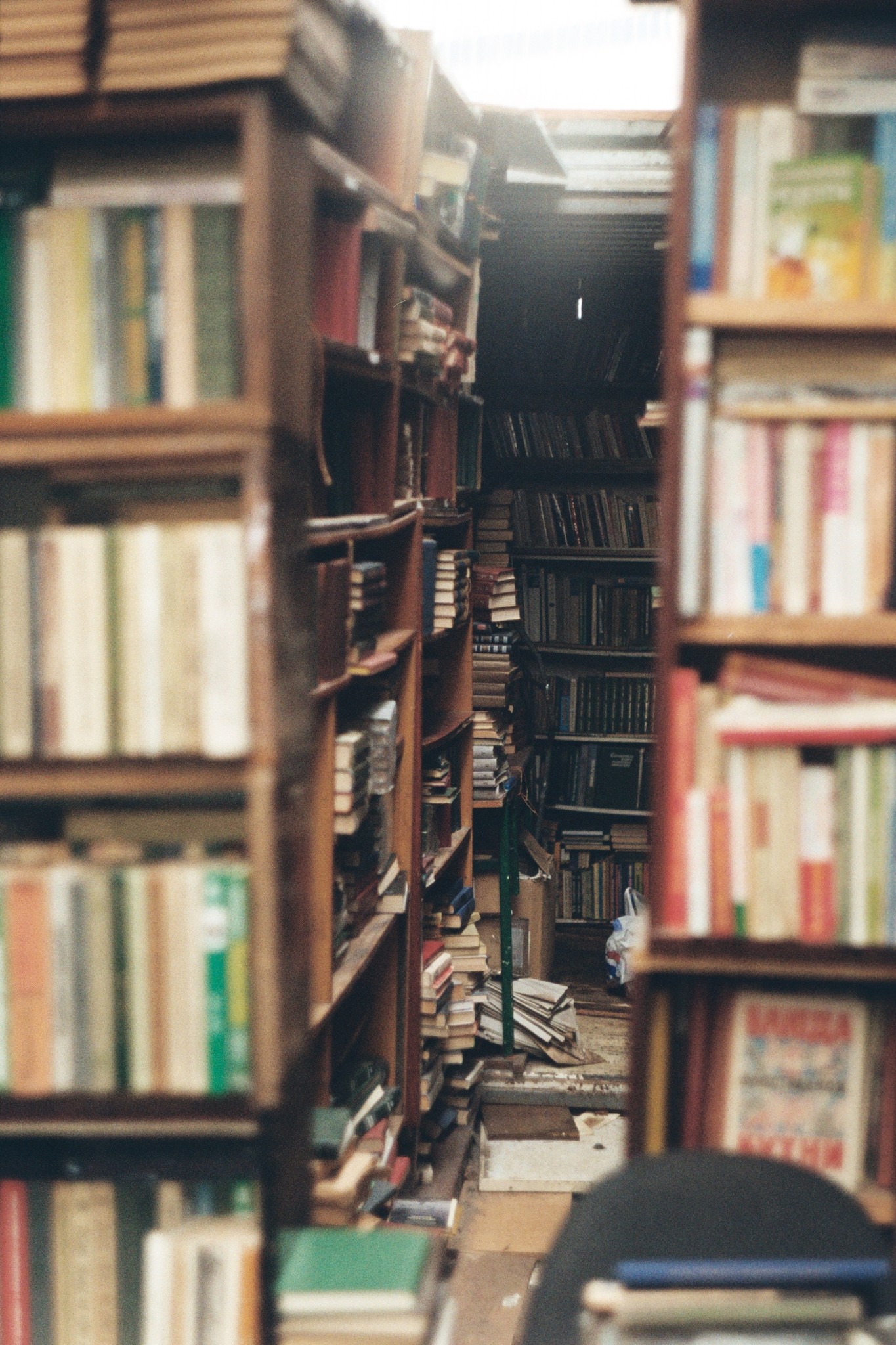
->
[508,1148,888,1345]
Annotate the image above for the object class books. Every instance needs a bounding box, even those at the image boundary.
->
[1,0,896,1345]
[798,763,835,944]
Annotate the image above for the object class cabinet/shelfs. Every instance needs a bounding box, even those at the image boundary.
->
[0,0,895,1345]
